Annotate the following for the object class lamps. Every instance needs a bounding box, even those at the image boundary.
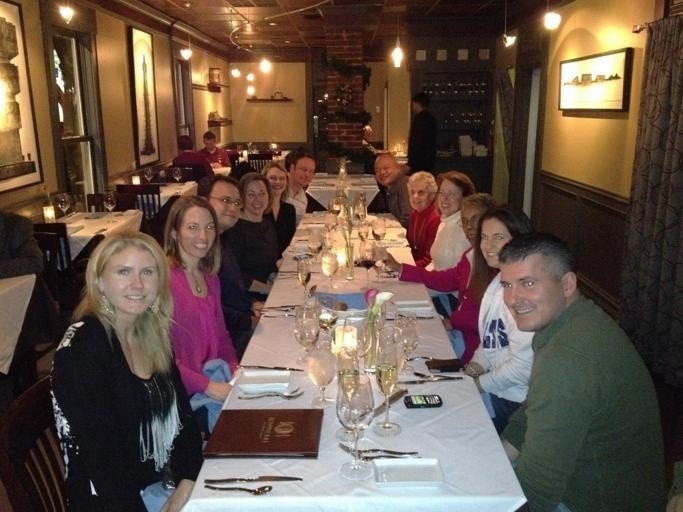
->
[502,0,516,47]
[543,0,561,30]
[389,13,405,68]
[179,35,193,60]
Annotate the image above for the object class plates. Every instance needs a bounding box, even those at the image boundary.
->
[394,300,432,311]
[372,458,445,487]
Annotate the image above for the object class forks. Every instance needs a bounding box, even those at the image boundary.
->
[238,391,303,402]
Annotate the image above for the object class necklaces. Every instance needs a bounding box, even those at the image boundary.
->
[191,270,201,293]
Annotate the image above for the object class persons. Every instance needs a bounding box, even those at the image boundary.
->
[380,193,503,365]
[238,173,283,302]
[406,93,437,173]
[163,194,240,435]
[286,153,316,214]
[362,126,384,157]
[171,135,214,173]
[0,214,65,344]
[51,230,204,510]
[261,162,297,268]
[197,131,232,167]
[370,154,413,228]
[465,203,535,428]
[198,174,261,359]
[498,234,668,511]
[427,170,473,299]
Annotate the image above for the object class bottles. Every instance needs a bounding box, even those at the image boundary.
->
[422,77,486,131]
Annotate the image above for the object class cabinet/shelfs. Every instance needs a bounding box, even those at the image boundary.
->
[418,70,489,145]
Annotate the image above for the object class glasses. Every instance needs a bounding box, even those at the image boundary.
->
[210,196,244,209]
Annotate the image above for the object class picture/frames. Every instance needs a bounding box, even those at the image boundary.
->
[0,0,44,195]
[557,47,633,112]
[127,25,160,169]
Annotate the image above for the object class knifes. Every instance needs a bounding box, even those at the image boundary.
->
[235,364,304,373]
[395,377,464,384]
[384,316,433,320]
[204,475,304,482]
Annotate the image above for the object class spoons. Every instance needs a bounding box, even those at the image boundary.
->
[204,483,273,498]
[413,370,451,380]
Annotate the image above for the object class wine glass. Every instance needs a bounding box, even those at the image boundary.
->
[103,191,119,224]
[260,158,419,478]
[144,167,154,184]
[55,193,72,225]
[212,144,282,170]
[173,167,183,191]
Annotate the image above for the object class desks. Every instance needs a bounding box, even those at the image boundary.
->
[236,149,290,169]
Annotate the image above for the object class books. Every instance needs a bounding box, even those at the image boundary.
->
[204,408,323,458]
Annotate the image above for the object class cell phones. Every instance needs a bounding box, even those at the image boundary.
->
[404,395,442,408]
